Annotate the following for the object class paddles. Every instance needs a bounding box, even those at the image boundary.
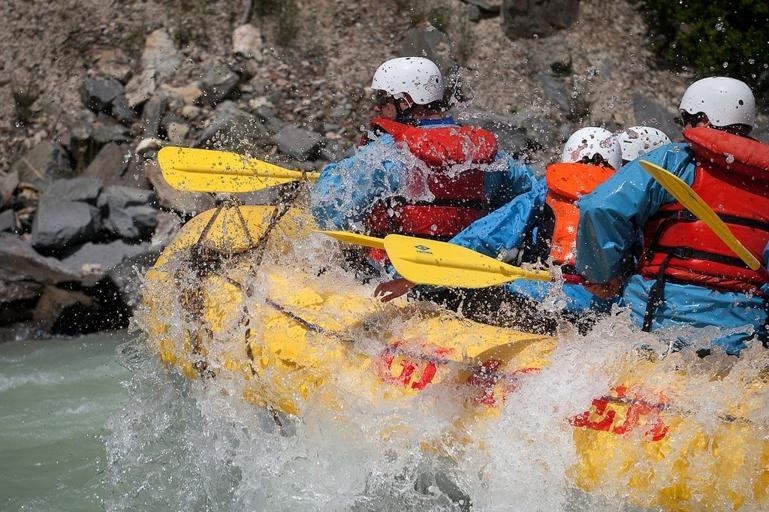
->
[637,160,769,283]
[384,235,583,290]
[157,146,323,192]
[311,230,384,249]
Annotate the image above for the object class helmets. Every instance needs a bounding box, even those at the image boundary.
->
[677,75,756,132]
[370,55,444,108]
[560,126,673,171]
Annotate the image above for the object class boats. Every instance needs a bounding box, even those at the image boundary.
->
[136,205,767,510]
